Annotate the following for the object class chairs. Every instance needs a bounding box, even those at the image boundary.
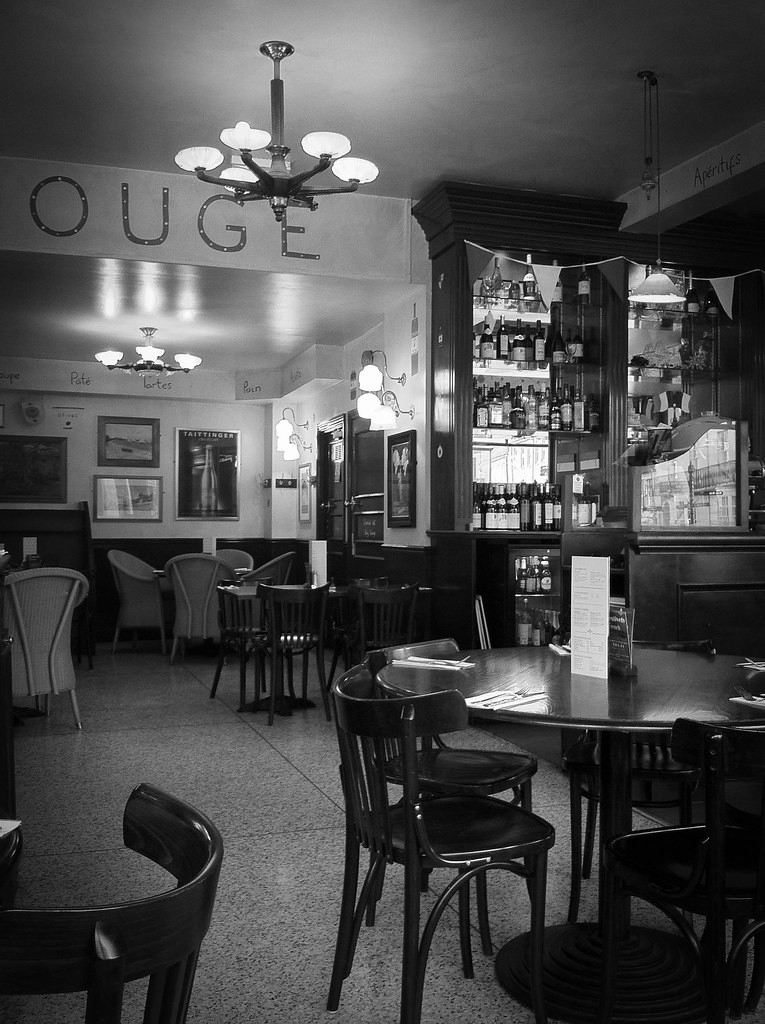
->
[0,545,765,1024]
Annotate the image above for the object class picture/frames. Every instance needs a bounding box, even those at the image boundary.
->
[386,429,417,529]
[93,474,164,523]
[0,434,68,503]
[296,463,312,525]
[175,427,242,522]
[97,415,160,468]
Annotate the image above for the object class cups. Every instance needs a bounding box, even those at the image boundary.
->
[701,410,719,417]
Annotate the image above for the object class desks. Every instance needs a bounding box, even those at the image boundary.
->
[213,580,432,720]
[374,643,765,1024]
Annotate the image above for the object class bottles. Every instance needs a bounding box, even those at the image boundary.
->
[510,484,521,513]
[515,556,537,594]
[411,303,418,376]
[542,482,562,531]
[532,608,545,647]
[552,260,563,301]
[491,257,503,297]
[482,482,497,529]
[578,482,592,527]
[496,485,512,513]
[544,609,562,647]
[686,271,720,317]
[534,556,552,594]
[201,444,218,510]
[519,482,534,531]
[532,482,545,531]
[523,253,536,299]
[472,380,600,432]
[350,370,356,400]
[472,315,583,364]
[516,597,532,645]
[572,493,578,527]
[473,481,484,531]
[578,261,590,305]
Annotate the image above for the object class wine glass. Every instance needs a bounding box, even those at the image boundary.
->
[482,273,511,298]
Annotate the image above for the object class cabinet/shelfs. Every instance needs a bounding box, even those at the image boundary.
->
[408,179,765,662]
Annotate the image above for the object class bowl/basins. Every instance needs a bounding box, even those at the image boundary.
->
[601,514,628,529]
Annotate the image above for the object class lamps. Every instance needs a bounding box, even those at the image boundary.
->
[276,407,309,437]
[627,70,688,302]
[369,390,415,431]
[173,40,380,222]
[283,433,313,461]
[277,432,306,452]
[358,349,406,392]
[93,327,203,378]
[356,383,384,418]
